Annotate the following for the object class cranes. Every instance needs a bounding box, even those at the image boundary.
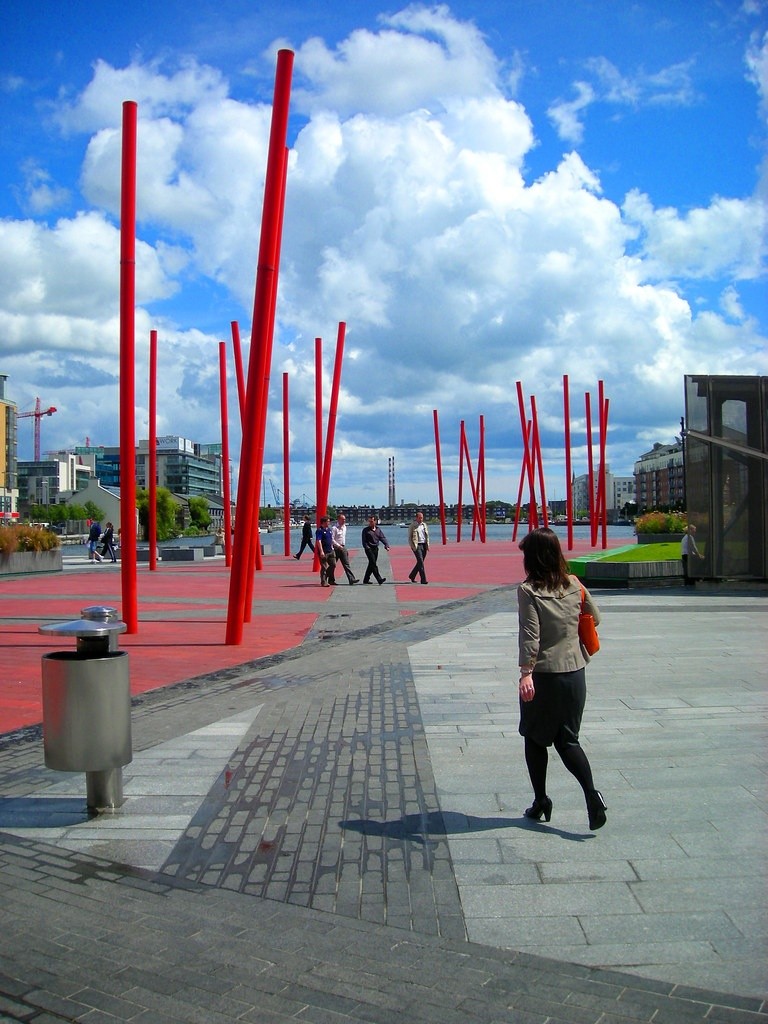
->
[15,397,57,462]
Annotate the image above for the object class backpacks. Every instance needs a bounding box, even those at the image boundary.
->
[92,520,101,537]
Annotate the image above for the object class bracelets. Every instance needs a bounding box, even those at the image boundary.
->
[520,669,533,674]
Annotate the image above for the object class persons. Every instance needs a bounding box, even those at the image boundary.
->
[408,512,430,584]
[362,514,391,585]
[293,515,315,560]
[316,516,339,587]
[680,524,704,591]
[214,527,225,555]
[325,514,360,585]
[85,517,121,565]
[518,527,608,830]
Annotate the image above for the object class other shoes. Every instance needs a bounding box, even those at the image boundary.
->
[348,579,359,584]
[378,578,386,584]
[421,581,428,584]
[293,555,300,560]
[408,575,417,583]
[328,582,338,585]
[322,583,330,587]
[363,581,373,584]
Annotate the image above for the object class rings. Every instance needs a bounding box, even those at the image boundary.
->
[527,688,532,691]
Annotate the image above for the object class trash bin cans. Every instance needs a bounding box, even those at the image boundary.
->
[38,607,133,823]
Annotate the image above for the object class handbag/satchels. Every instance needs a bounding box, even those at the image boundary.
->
[577,612,600,656]
[100,535,106,543]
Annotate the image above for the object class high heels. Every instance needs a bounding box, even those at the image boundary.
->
[585,789,608,830]
[524,796,553,822]
